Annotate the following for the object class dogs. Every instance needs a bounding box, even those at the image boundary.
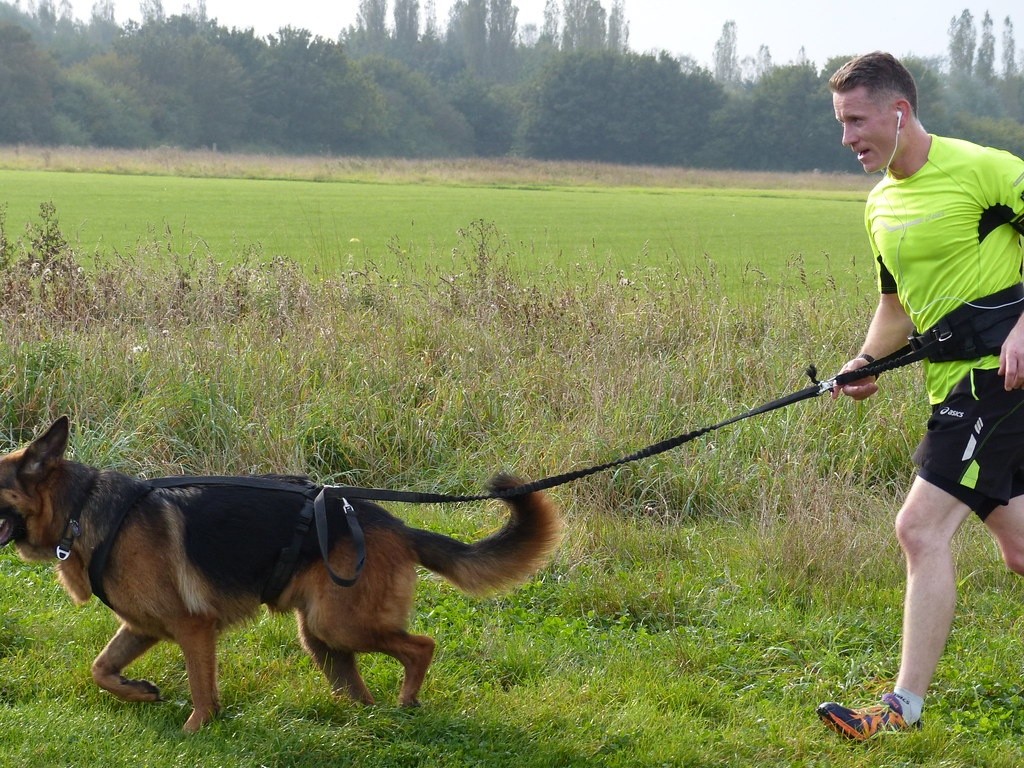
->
[0,417,564,731]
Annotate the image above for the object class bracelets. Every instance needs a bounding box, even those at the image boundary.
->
[858,352,883,383]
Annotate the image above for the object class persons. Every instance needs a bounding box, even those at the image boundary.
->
[808,47,1024,745]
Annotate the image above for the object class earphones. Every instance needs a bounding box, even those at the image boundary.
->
[895,111,903,129]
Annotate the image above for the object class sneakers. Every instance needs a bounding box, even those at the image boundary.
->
[816,692,922,745]
